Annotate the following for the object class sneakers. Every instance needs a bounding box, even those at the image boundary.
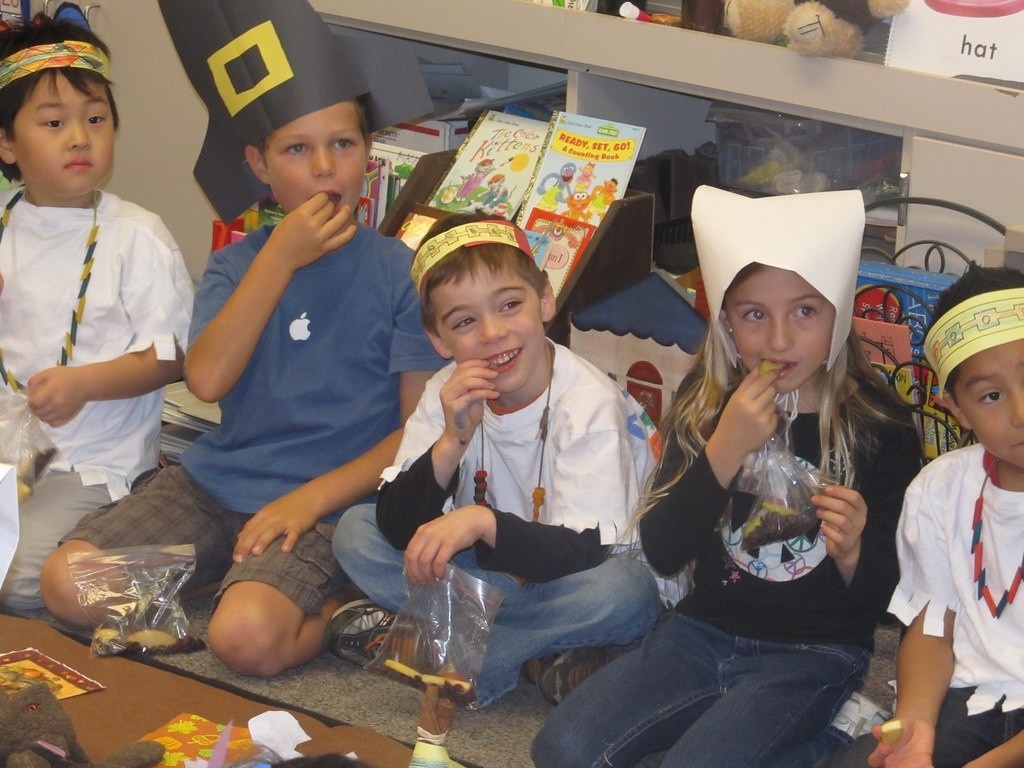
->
[832,690,894,739]
[324,597,431,680]
[521,645,619,707]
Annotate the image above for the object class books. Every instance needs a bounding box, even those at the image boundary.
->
[211,105,648,297]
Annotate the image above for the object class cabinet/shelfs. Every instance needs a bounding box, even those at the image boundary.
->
[305,0,1024,277]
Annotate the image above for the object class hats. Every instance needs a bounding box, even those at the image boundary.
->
[157,0,434,227]
[690,184,865,370]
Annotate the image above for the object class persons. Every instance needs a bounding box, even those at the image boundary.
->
[531,185,929,768]
[41,92,456,678]
[325,214,690,708]
[0,18,196,620]
[822,260,1024,768]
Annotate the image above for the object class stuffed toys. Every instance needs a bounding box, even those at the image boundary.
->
[0,682,164,768]
[725,0,908,60]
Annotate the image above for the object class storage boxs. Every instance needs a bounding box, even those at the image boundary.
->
[715,122,901,206]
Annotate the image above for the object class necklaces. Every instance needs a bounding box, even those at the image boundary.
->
[971,458,1024,619]
[473,341,553,523]
[0,188,99,394]
